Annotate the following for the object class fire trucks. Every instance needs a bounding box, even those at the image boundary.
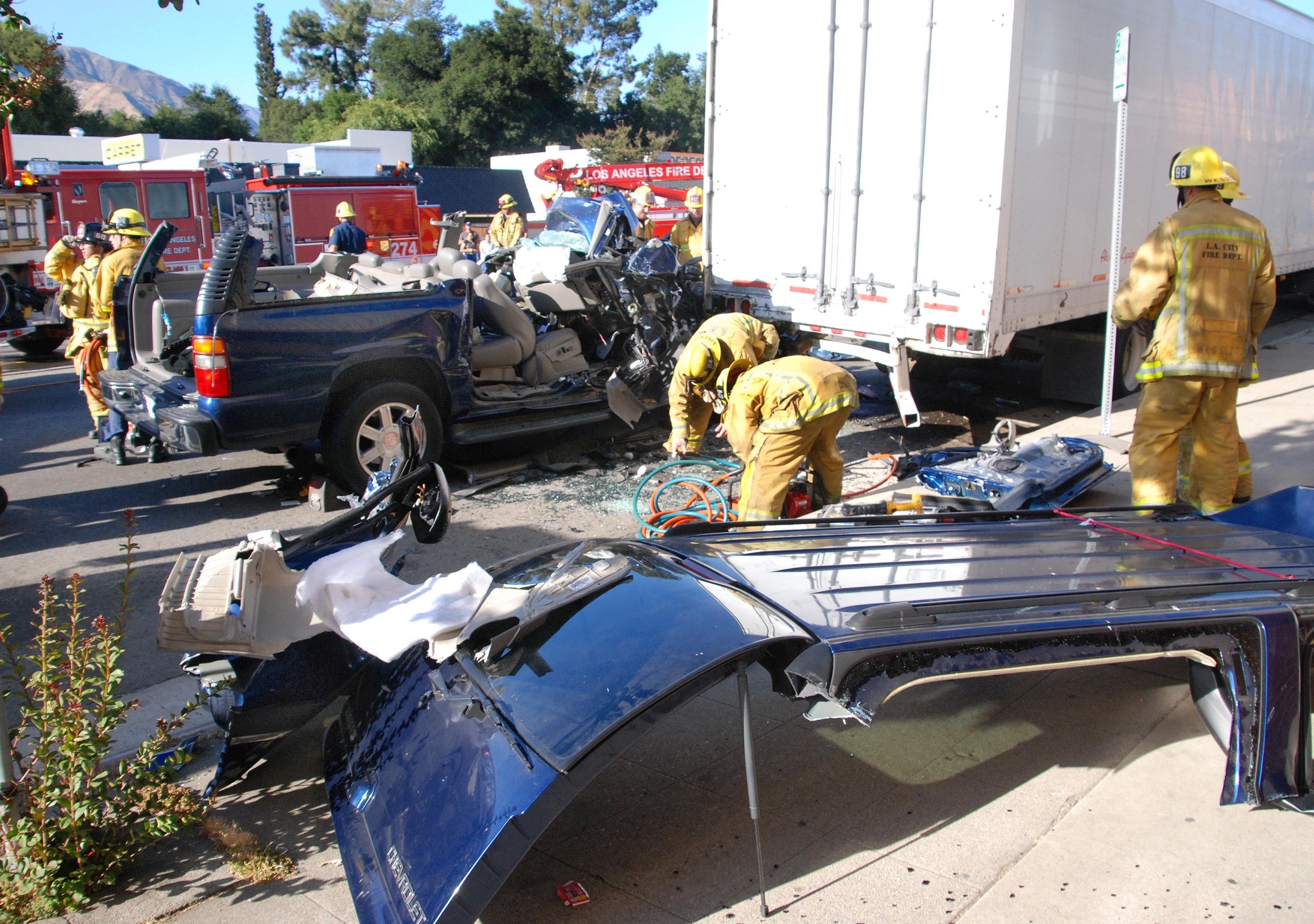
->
[1,118,690,353]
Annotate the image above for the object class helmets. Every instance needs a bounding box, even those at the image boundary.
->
[498,194,517,208]
[1213,162,1250,199]
[683,186,703,209]
[102,208,152,236]
[626,186,655,208]
[716,359,754,406]
[335,201,356,218]
[76,222,110,246]
[679,333,721,386]
[1166,144,1238,188]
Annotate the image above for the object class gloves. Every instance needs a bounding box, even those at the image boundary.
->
[62,234,78,249]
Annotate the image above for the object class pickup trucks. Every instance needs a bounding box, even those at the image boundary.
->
[98,220,703,500]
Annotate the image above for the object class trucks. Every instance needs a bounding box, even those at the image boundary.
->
[702,0,1313,433]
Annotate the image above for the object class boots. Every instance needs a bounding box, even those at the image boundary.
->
[110,435,126,466]
[147,436,169,463]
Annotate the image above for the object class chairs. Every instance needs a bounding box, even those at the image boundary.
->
[348,247,601,384]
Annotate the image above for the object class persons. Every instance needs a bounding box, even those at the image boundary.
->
[44,222,135,439]
[1176,161,1260,503]
[670,185,703,277]
[627,186,659,247]
[93,208,168,465]
[716,355,859,532]
[663,312,780,466]
[329,202,367,254]
[458,222,494,270]
[522,227,528,238]
[1111,145,1276,515]
[490,194,524,248]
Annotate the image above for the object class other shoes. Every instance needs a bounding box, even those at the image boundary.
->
[88,429,98,439]
[667,450,699,467]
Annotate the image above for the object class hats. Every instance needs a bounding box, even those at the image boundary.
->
[465,222,471,225]
